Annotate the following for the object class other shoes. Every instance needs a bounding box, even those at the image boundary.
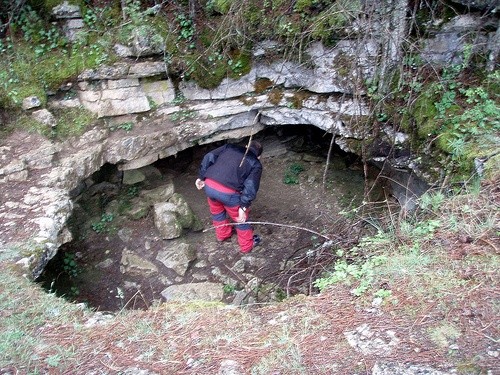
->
[218,226,235,242]
[246,234,260,253]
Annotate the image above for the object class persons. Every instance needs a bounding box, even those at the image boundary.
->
[195,140,264,255]
